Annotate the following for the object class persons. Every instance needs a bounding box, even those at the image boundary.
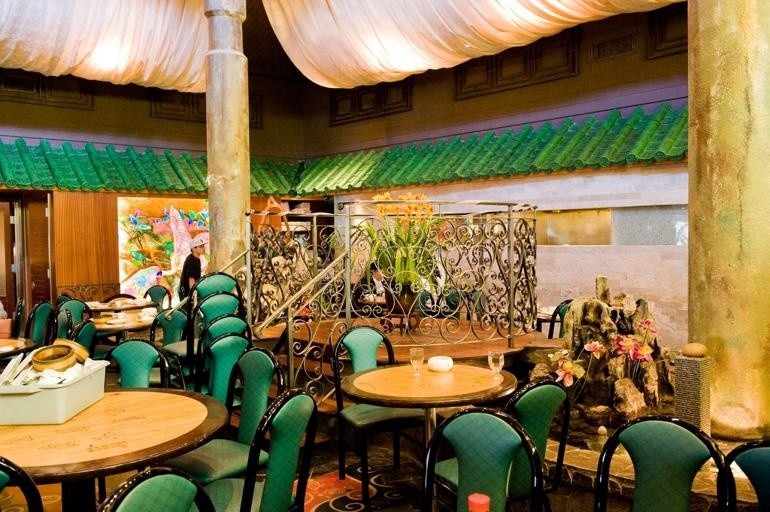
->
[178,238,209,312]
[356,260,385,303]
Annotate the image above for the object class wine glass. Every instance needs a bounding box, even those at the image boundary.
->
[409,346,424,377]
[488,352,506,379]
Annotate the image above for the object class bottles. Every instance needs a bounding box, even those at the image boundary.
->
[467,492,490,511]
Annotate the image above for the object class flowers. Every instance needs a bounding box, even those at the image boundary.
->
[350,194,447,293]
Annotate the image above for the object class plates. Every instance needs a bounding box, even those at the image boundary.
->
[106,313,132,325]
[138,312,159,323]
[0,349,37,388]
[86,298,149,308]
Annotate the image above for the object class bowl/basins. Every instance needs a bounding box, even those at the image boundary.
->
[93,314,113,323]
[33,334,91,372]
[141,307,158,316]
[428,355,453,371]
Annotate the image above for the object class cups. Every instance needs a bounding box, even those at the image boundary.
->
[37,357,96,388]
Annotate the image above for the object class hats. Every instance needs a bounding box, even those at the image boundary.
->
[190,239,208,250]
